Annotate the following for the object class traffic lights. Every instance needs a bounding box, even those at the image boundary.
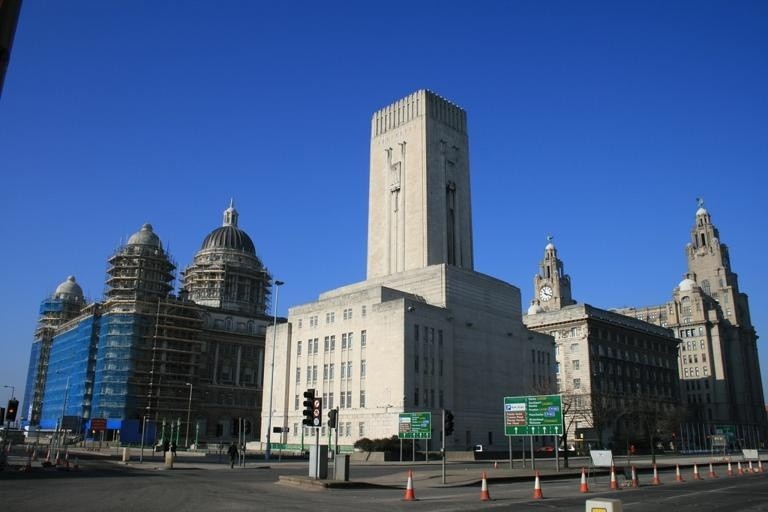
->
[312,398,322,428]
[443,410,454,437]
[5,400,19,423]
[327,409,336,429]
[244,421,251,435]
[302,389,315,427]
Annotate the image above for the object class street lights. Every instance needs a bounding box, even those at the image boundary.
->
[3,385,15,450]
[183,382,193,449]
[54,369,70,449]
[263,280,284,462]
[89,413,201,465]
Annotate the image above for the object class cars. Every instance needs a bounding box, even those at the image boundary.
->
[469,442,577,455]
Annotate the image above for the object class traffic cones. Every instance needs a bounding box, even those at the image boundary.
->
[532,471,543,500]
[608,463,618,490]
[5,440,81,475]
[399,468,420,502]
[492,461,497,469]
[479,471,494,501]
[628,457,764,490]
[579,465,589,493]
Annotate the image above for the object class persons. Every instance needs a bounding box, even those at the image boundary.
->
[164,438,178,456]
[227,441,238,469]
